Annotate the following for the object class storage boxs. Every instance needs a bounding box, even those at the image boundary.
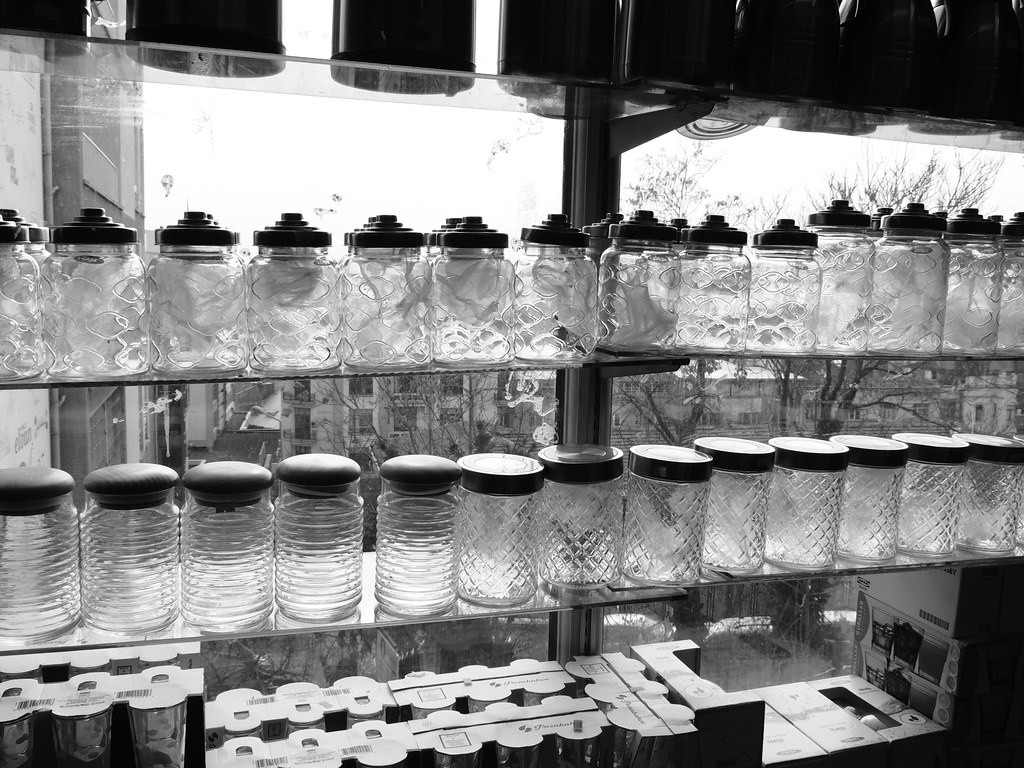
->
[727,564,1024,768]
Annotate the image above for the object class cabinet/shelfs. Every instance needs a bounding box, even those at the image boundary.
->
[0,28,1024,768]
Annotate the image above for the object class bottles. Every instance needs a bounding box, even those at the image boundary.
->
[765,436,849,568]
[81,463,180,638]
[693,437,776,574]
[273,453,364,625]
[538,444,624,589]
[623,444,713,584]
[0,466,82,643]
[582,211,749,351]
[951,434,1024,553]
[830,435,910,561]
[180,461,274,633]
[747,218,822,354]
[374,454,461,617]
[891,433,970,555]
[456,453,545,606]
[806,200,1024,358]
[0,207,598,379]
